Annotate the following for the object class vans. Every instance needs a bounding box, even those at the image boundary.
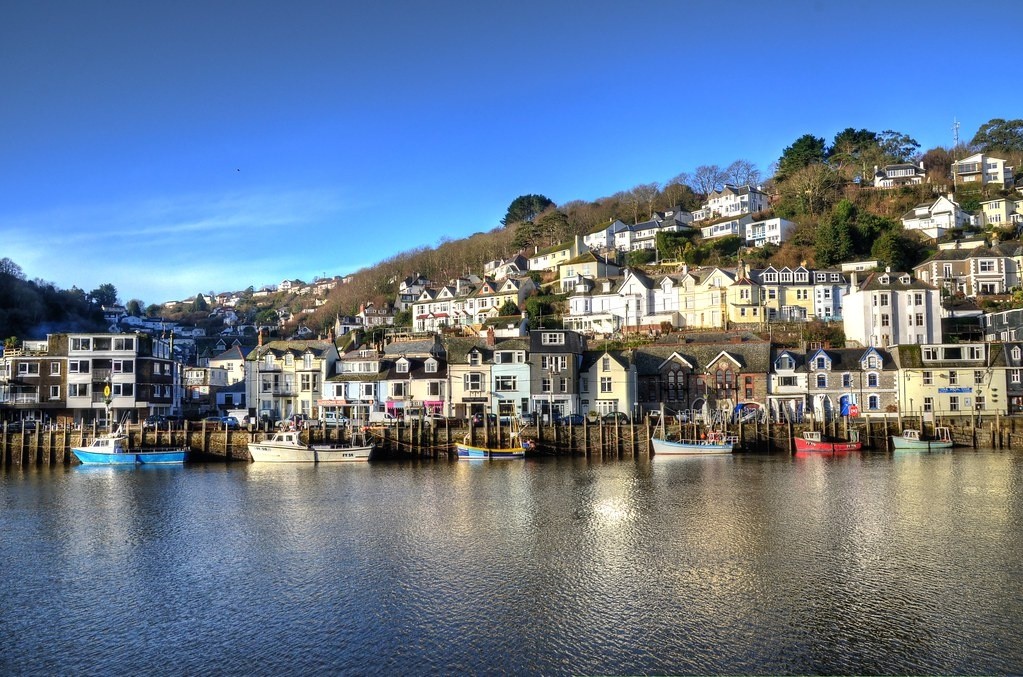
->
[318,412,349,428]
[369,412,401,427]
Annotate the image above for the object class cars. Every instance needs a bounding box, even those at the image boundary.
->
[473,413,534,428]
[220,417,240,430]
[558,414,590,426]
[206,417,221,422]
[240,415,249,430]
[1,421,36,433]
[275,418,290,428]
[602,412,629,425]
[143,415,180,431]
[424,414,447,427]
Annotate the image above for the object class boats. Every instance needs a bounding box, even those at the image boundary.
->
[891,427,953,449]
[247,404,375,463]
[455,414,526,459]
[649,399,757,425]
[70,411,191,464]
[650,404,739,454]
[794,432,862,452]
